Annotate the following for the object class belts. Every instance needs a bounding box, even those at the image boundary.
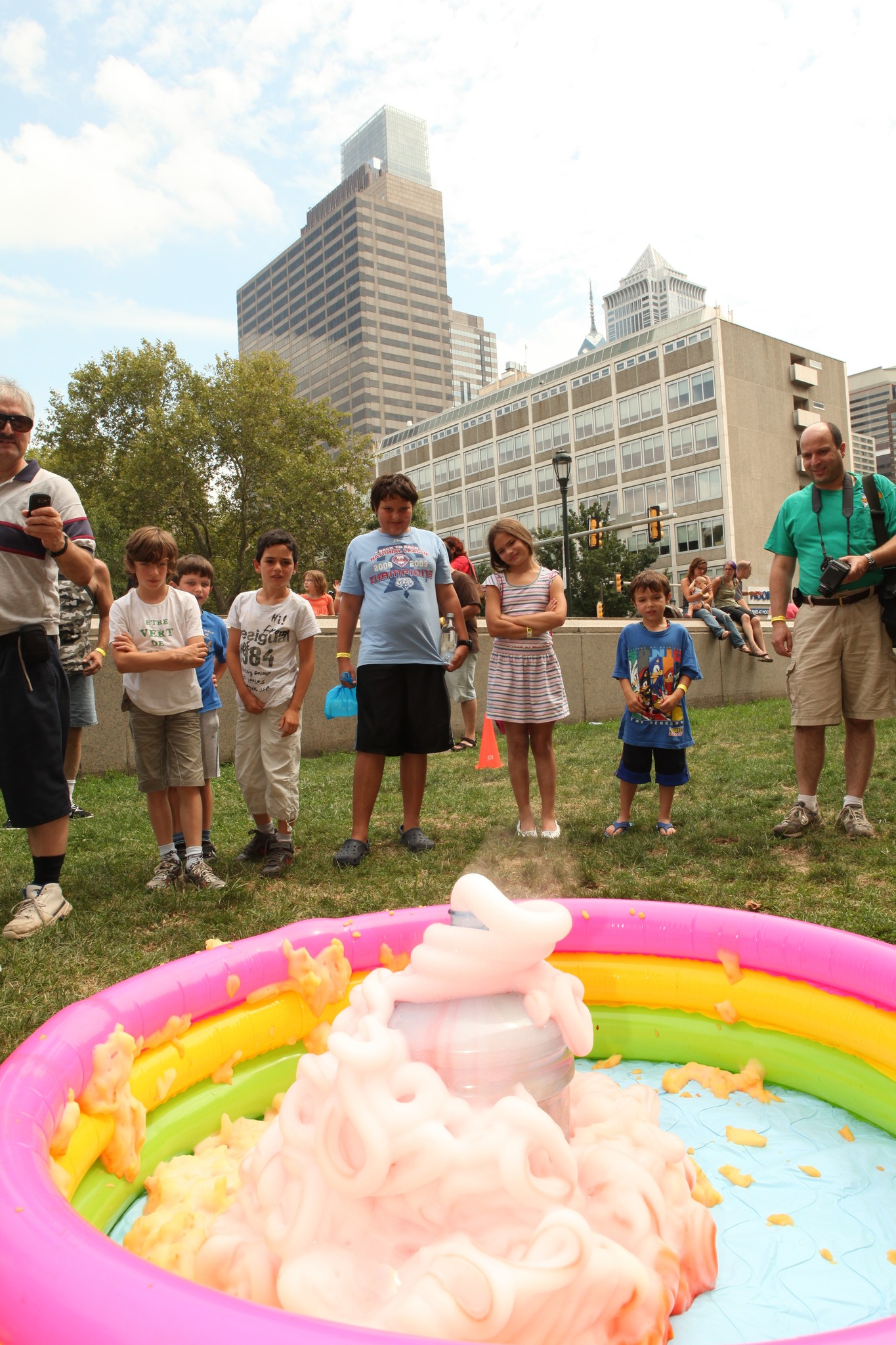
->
[801,584,882,605]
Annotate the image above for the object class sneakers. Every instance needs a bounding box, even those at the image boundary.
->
[263,840,295,876]
[145,836,226,892]
[833,804,876,840]
[237,828,278,863]
[2,883,73,940]
[0,816,22,830]
[773,801,826,837]
[68,803,94,821]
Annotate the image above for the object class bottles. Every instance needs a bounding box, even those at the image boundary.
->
[440,613,458,664]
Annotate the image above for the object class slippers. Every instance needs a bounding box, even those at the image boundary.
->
[718,631,772,662]
[656,822,676,837]
[605,822,633,837]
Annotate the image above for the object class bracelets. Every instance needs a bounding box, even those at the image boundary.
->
[95,648,106,658]
[526,627,532,638]
[700,592,704,595]
[677,684,687,694]
[699,601,704,606]
[48,531,68,557]
[336,653,351,659]
[748,591,750,595]
[441,617,445,626]
[771,616,786,624]
[335,596,341,599]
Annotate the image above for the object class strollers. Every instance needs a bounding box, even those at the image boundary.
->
[663,604,685,618]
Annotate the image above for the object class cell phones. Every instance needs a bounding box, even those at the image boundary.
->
[28,492,51,526]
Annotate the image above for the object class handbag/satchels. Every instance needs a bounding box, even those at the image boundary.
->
[324,672,359,720]
[467,557,485,604]
[663,604,683,618]
[862,472,896,648]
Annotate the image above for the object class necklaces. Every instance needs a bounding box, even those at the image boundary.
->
[639,617,670,631]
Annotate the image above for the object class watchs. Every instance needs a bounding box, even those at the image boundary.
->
[458,640,472,651]
[863,553,876,572]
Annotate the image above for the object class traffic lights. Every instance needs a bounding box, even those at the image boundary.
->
[614,572,624,594]
[587,516,602,551]
[596,603,605,618]
[647,505,664,543]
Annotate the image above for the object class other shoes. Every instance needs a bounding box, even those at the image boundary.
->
[541,820,560,839]
[517,820,538,838]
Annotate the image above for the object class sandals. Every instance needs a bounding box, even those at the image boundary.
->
[451,737,478,751]
[332,838,370,867]
[399,825,435,853]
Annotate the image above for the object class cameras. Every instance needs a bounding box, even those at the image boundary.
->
[816,555,851,598]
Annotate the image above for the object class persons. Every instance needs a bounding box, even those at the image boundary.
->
[764,422,896,840]
[57,474,481,894]
[604,570,704,837]
[680,558,799,662]
[0,377,95,941]
[480,519,570,839]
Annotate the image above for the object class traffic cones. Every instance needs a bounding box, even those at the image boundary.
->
[474,712,504,770]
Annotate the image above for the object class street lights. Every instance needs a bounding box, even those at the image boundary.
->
[551,446,572,617]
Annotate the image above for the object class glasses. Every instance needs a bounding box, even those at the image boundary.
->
[0,413,33,433]
[741,561,751,570]
[723,568,734,571]
[302,579,315,583]
[697,566,706,572]
